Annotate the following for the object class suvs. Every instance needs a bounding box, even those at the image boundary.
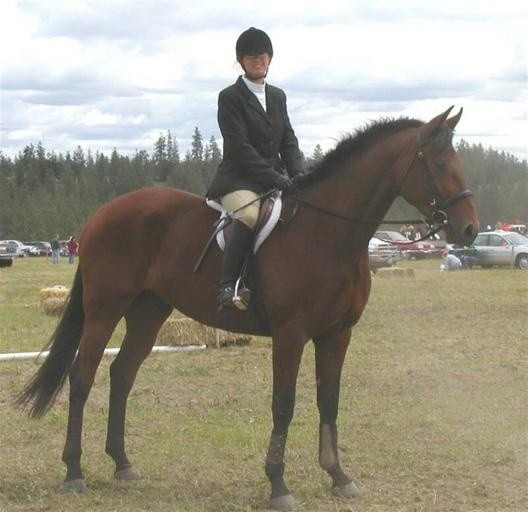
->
[0,240,40,257]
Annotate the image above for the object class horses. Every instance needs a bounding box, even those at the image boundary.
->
[8,105,480,512]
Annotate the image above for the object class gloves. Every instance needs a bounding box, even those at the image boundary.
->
[274,174,292,190]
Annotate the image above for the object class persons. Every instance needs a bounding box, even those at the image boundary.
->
[50,233,62,264]
[67,237,77,264]
[205,27,308,316]
[400,221,503,271]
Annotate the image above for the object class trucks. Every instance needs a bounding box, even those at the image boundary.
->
[0,243,18,267]
[57,241,79,256]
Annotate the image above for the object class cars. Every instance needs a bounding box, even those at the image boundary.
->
[367,237,402,274]
[441,230,527,270]
[374,231,438,260]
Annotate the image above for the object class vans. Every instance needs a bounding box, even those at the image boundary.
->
[493,225,526,234]
[23,241,52,255]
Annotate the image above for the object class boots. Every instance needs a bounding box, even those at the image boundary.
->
[215,219,252,314]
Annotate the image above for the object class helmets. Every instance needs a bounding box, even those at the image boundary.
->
[236,27,272,57]
